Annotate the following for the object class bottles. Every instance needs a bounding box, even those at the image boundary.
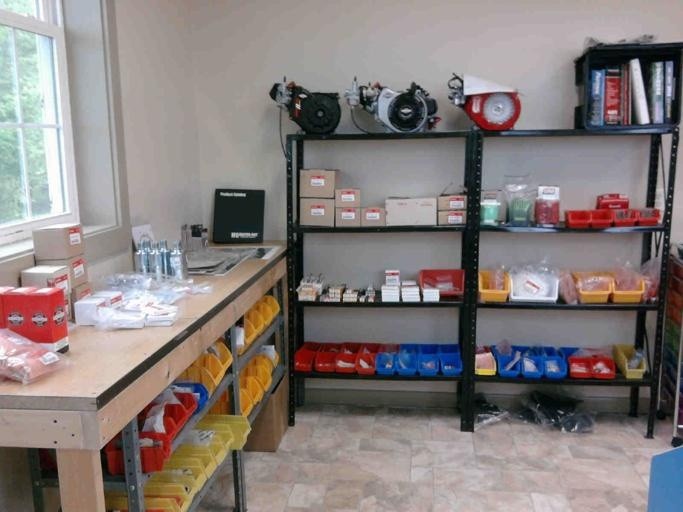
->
[134,238,188,280]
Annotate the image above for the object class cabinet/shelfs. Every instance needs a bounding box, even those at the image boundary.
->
[286,126,681,441]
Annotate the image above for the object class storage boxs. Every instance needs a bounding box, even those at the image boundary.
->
[18,223,124,328]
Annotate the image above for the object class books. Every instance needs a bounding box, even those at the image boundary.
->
[589,58,674,125]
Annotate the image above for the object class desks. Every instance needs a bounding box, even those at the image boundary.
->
[0,240,290,512]
